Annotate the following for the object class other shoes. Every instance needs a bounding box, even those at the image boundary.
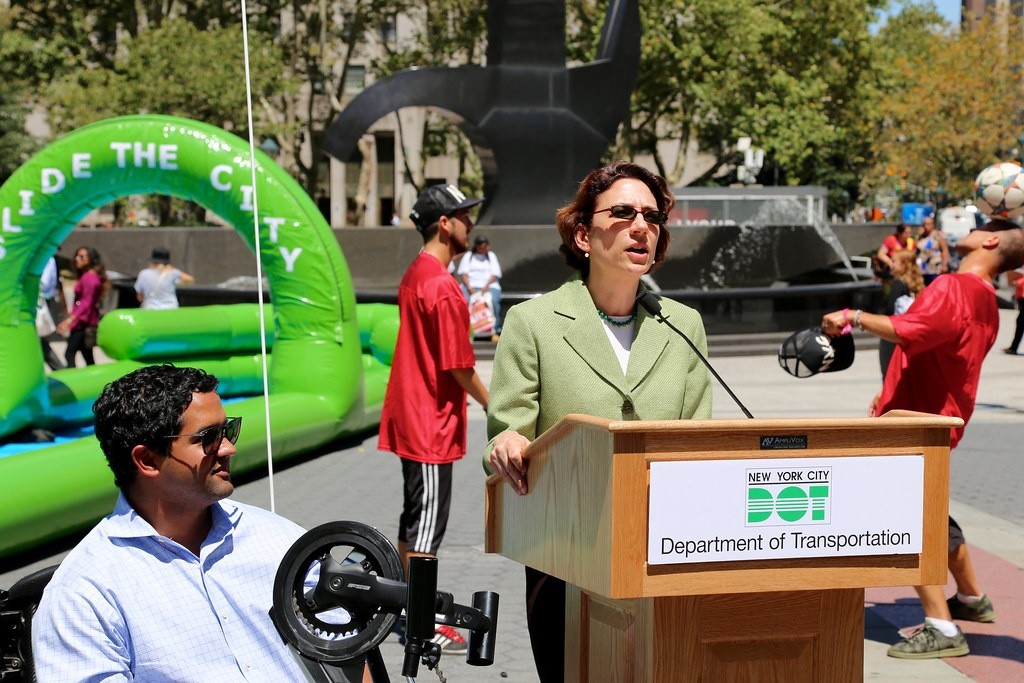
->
[491,334,500,342]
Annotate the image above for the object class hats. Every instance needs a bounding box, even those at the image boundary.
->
[409,183,486,230]
[778,324,856,378]
[149,246,171,264]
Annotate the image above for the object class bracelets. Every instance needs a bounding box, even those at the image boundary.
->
[855,310,865,331]
[840,309,853,334]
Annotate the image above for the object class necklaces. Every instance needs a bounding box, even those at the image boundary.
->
[597,309,637,327]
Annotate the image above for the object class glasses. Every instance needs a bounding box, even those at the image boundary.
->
[591,205,668,225]
[163,416,242,457]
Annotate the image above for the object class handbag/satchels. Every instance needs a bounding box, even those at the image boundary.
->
[468,291,496,334]
[84,325,99,348]
[872,257,893,278]
[33,296,57,337]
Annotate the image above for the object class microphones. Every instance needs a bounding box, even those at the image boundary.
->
[633,290,754,418]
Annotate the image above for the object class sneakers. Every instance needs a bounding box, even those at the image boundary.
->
[888,619,970,659]
[430,624,469,656]
[947,593,995,623]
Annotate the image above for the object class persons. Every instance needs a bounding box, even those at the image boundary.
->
[57,246,109,368]
[457,235,502,341]
[31,362,375,683]
[37,256,63,369]
[134,247,193,307]
[878,216,949,285]
[1004,267,1024,354]
[821,219,1024,659]
[484,161,712,683]
[377,184,488,654]
[880,249,926,380]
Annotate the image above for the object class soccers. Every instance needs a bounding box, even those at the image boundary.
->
[973,162,1024,219]
